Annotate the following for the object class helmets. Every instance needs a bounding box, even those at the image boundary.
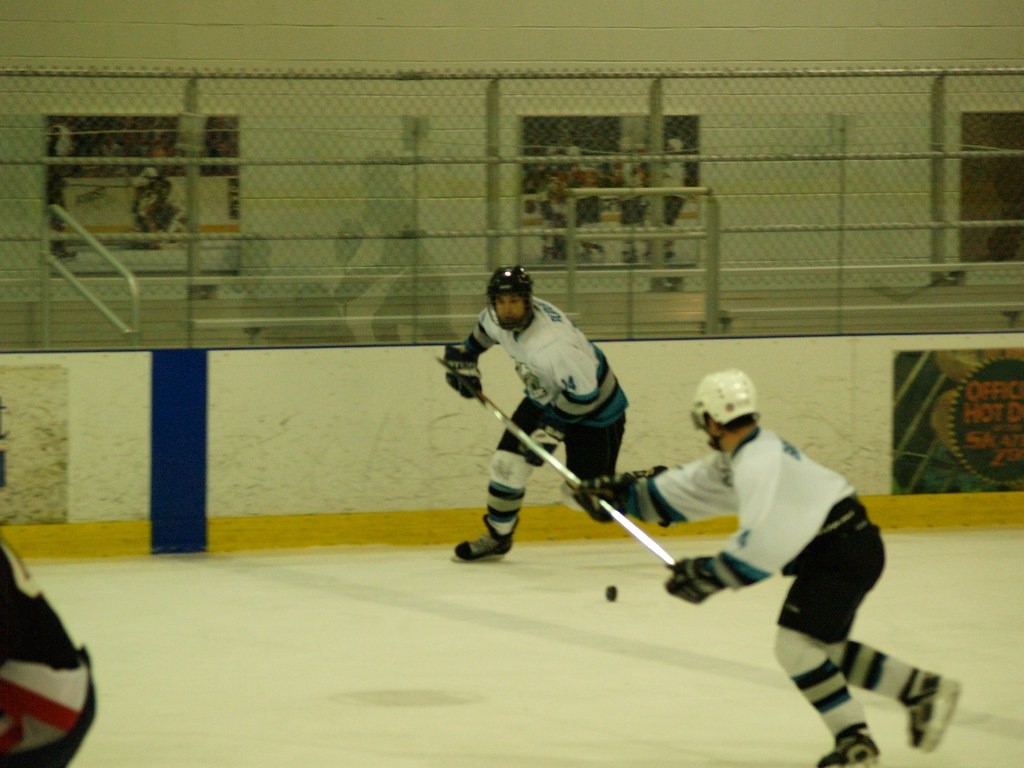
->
[486,266,533,301]
[692,368,761,432]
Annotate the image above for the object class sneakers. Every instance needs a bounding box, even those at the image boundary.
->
[904,671,962,751]
[816,735,879,768]
[450,513,519,565]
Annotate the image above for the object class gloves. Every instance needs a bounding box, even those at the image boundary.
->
[574,466,632,522]
[444,345,482,399]
[518,420,565,466]
[666,556,744,603]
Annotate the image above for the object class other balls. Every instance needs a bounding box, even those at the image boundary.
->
[605,586,617,602]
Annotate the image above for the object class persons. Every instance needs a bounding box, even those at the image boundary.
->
[0,531,96,768]
[533,127,690,267]
[443,265,671,562]
[572,369,961,768]
[46,122,240,259]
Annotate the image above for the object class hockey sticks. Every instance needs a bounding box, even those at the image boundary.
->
[437,356,582,486]
[559,477,680,573]
